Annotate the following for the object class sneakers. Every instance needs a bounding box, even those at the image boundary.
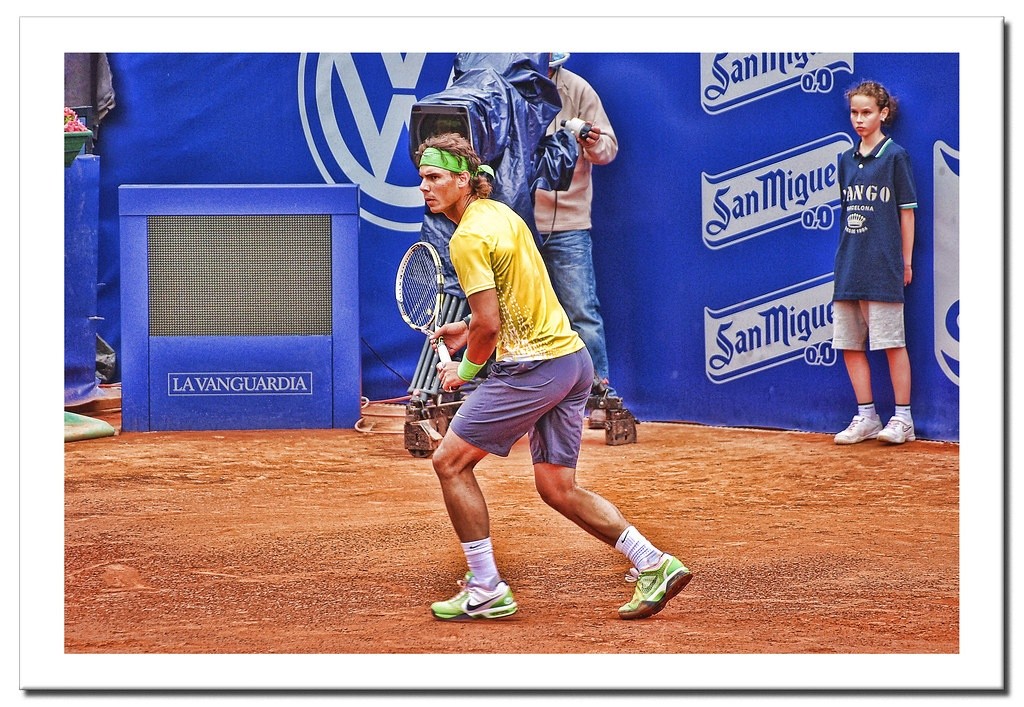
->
[878,416,915,443]
[617,553,693,621]
[431,572,518,621]
[834,414,883,445]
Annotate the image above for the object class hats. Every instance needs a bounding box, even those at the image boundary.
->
[549,53,570,67]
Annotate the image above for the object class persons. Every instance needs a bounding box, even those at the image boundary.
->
[414,132,693,621]
[534,52,618,428]
[831,80,918,444]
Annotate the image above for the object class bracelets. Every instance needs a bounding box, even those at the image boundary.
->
[457,348,487,382]
[463,315,470,330]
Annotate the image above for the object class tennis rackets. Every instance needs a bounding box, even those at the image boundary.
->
[391,241,463,392]
